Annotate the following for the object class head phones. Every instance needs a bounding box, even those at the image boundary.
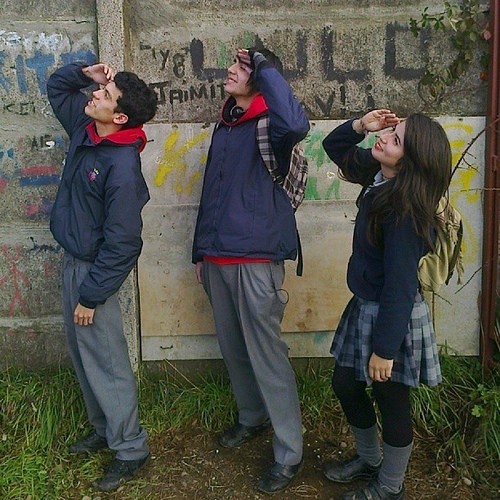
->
[230,105,247,118]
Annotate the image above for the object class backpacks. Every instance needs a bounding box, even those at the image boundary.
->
[417,195,464,294]
[256,116,308,212]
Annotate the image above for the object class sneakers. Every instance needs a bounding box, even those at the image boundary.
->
[324,454,384,483]
[344,483,404,500]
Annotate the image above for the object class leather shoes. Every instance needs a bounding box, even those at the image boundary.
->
[258,458,304,493]
[219,418,275,448]
[68,429,110,456]
[99,451,151,491]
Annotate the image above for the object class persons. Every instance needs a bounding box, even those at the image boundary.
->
[322,108,454,500]
[46,62,159,490]
[191,46,311,494]
